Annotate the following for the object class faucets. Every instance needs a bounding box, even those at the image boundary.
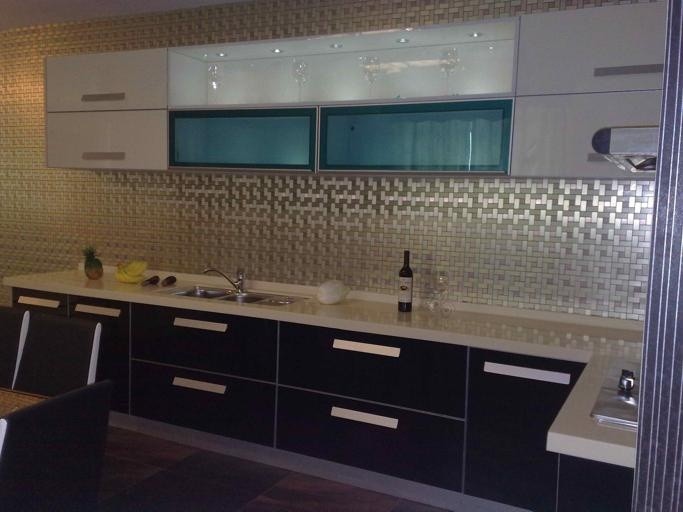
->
[201,266,245,290]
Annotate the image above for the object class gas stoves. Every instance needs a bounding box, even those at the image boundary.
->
[587,357,643,428]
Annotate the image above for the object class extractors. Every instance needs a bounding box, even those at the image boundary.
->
[591,124,662,171]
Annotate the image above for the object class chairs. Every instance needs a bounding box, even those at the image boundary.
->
[0,306,119,512]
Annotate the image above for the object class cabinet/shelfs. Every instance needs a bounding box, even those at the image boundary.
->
[511,0,676,182]
[169,17,518,180]
[130,304,277,449]
[44,48,170,171]
[9,288,131,416]
[277,320,468,494]
[467,346,588,510]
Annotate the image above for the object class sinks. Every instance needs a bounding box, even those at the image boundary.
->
[220,292,267,303]
[173,288,227,298]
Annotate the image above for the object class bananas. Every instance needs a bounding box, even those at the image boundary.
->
[113,260,148,283]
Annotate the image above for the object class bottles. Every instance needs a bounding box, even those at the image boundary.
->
[397,251,413,313]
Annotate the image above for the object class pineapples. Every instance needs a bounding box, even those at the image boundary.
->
[79,246,103,280]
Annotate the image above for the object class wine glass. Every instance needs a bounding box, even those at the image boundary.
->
[431,271,449,320]
